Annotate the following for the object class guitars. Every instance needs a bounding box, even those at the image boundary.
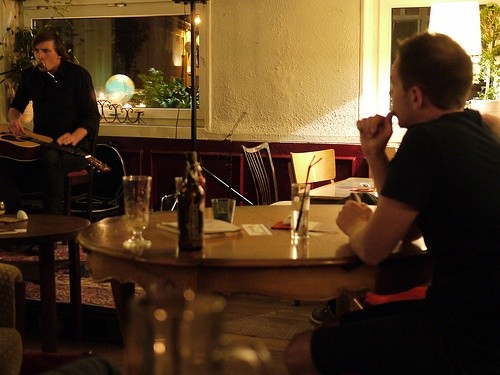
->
[0,121,109,174]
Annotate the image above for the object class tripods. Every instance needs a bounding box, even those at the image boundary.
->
[169,1,253,212]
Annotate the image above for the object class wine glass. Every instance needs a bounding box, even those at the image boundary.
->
[122,175,153,250]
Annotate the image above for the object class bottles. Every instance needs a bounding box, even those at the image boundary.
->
[178,151,205,252]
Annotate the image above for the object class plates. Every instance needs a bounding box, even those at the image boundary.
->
[162,217,241,233]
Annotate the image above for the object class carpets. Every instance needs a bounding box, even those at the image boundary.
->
[1,244,146,310]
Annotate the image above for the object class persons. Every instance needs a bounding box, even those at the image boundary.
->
[0,30,102,216]
[310,32,500,375]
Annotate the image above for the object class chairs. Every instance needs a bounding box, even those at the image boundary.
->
[291,149,336,184]
[240,141,292,206]
[69,144,125,221]
[0,264,24,375]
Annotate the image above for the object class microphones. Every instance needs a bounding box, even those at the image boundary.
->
[19,59,37,72]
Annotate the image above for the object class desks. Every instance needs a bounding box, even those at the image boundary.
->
[310,177,375,205]
[76,205,427,375]
[0,214,90,354]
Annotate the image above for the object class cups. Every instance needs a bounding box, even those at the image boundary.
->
[210,198,236,224]
[130,291,272,375]
[291,183,310,241]
